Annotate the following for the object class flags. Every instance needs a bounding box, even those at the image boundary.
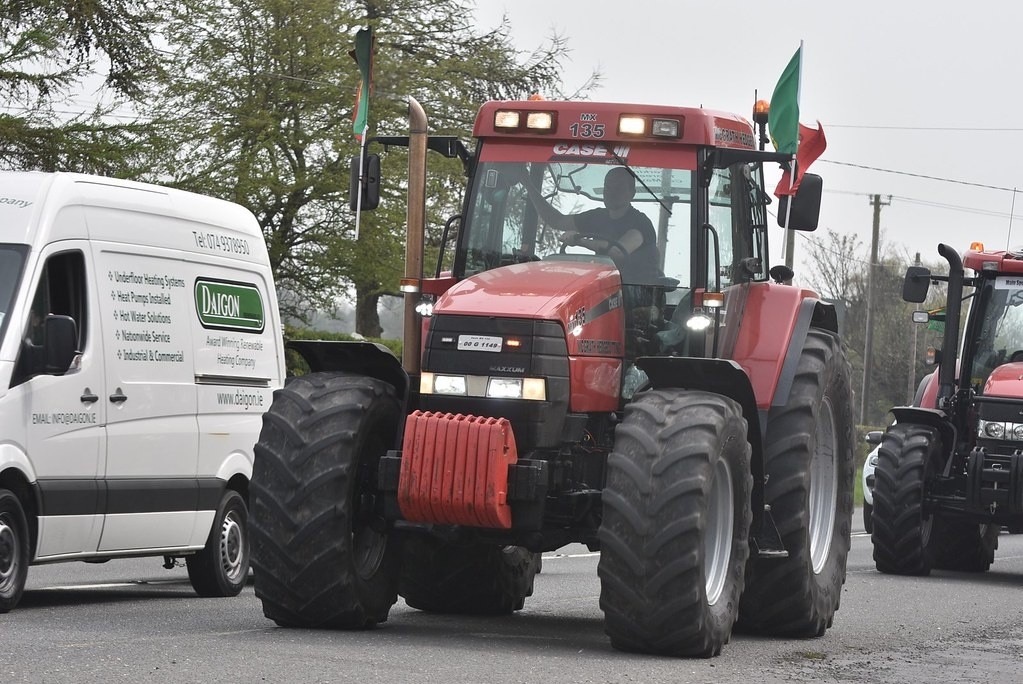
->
[927,308,947,336]
[350,27,375,140]
[768,44,828,199]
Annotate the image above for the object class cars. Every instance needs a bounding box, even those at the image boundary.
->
[860,418,898,534]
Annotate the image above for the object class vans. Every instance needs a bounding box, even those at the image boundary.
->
[0,170,289,617]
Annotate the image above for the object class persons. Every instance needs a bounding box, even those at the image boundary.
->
[521,167,660,310]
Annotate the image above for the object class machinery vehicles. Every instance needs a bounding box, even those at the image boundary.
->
[243,26,861,660]
[868,242,1023,576]
